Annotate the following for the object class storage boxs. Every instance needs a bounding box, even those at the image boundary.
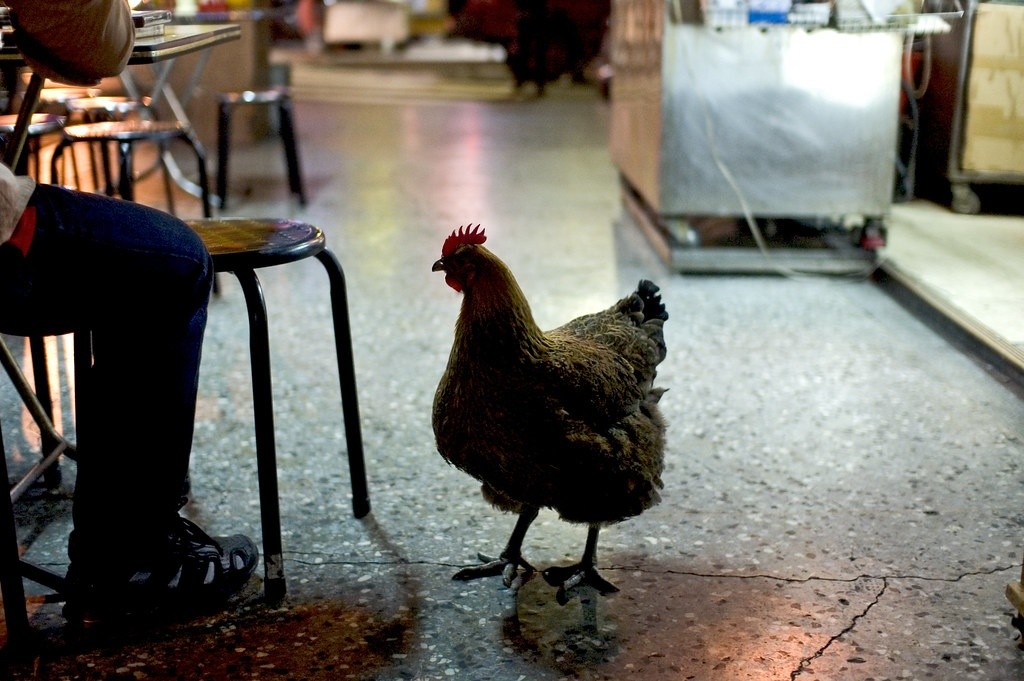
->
[698,1,831,30]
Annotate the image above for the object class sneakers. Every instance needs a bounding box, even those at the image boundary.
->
[67,514,261,616]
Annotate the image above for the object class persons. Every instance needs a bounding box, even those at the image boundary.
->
[0,1,259,612]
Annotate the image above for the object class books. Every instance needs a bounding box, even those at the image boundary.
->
[0,10,173,45]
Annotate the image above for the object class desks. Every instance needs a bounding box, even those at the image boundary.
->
[1,21,241,590]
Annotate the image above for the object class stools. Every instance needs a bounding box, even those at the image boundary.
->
[216,86,308,210]
[182,215,370,602]
[0,71,222,299]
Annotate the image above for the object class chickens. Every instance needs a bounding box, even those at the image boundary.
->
[429,223,668,606]
